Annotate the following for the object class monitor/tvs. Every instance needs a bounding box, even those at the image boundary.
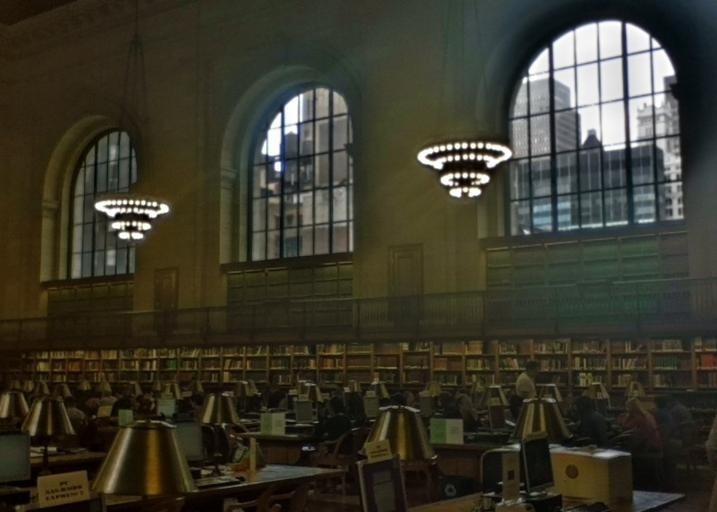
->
[363,396,381,419]
[294,399,314,422]
[489,403,507,431]
[171,418,205,464]
[427,416,465,446]
[158,397,177,417]
[521,429,556,494]
[0,430,33,485]
[353,453,408,512]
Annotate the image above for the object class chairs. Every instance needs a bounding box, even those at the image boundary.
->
[4,394,715,512]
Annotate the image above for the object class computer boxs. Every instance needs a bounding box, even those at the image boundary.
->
[480,491,563,512]
[117,409,133,426]
[0,490,30,512]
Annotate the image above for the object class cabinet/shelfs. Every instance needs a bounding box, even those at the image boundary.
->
[14,335,716,407]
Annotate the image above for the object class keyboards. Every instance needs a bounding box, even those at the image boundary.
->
[193,474,240,488]
[563,503,592,512]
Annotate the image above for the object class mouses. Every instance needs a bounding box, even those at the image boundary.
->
[235,476,245,482]
[591,499,608,511]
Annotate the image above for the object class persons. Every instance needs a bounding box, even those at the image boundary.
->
[64,361,717,484]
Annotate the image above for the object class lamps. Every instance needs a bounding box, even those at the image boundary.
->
[410,132,515,203]
[4,379,650,511]
[88,188,174,240]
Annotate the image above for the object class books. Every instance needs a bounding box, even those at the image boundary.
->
[7,337,716,390]
